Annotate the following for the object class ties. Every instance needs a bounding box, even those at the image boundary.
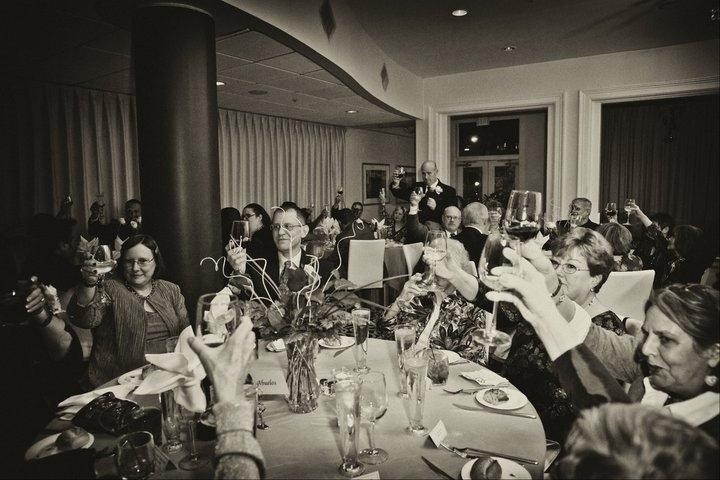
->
[279,262,292,286]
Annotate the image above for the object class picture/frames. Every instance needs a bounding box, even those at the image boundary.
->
[362,162,390,206]
[396,164,416,205]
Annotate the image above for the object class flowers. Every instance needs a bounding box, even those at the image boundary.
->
[212,213,422,411]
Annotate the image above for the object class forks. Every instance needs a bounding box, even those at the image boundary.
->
[453,449,527,468]
[441,442,540,465]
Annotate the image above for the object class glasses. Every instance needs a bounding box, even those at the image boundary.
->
[268,224,303,230]
[549,259,590,275]
[122,257,156,270]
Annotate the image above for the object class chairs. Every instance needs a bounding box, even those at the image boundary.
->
[331,231,661,335]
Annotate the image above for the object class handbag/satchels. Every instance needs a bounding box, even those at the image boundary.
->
[71,391,139,436]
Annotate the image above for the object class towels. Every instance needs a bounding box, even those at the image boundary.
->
[203,287,237,337]
[135,327,209,415]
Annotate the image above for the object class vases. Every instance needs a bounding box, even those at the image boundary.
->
[282,339,321,409]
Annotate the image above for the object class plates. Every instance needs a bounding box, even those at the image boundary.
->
[415,350,461,366]
[461,455,534,480]
[317,336,355,348]
[25,432,94,461]
[476,388,528,411]
[117,368,148,387]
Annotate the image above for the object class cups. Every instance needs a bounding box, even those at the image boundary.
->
[115,431,156,478]
[165,336,179,354]
[423,349,450,385]
[504,190,544,242]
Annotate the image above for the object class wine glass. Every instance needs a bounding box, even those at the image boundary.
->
[92,244,113,306]
[471,234,520,347]
[352,308,371,374]
[195,293,239,427]
[570,216,581,233]
[605,203,617,222]
[53,162,503,252]
[622,199,636,226]
[160,391,186,455]
[394,325,416,398]
[400,357,430,437]
[420,228,449,293]
[332,366,359,434]
[334,379,366,478]
[358,370,390,467]
[229,220,250,284]
[178,404,210,471]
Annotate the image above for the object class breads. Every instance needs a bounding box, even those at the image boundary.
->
[141,363,163,381]
[54,425,91,451]
[483,388,509,406]
[469,455,502,480]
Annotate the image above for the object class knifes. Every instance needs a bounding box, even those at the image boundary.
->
[453,402,538,420]
[334,344,355,358]
[421,456,452,478]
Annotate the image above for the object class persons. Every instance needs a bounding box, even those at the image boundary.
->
[1,160,719,479]
